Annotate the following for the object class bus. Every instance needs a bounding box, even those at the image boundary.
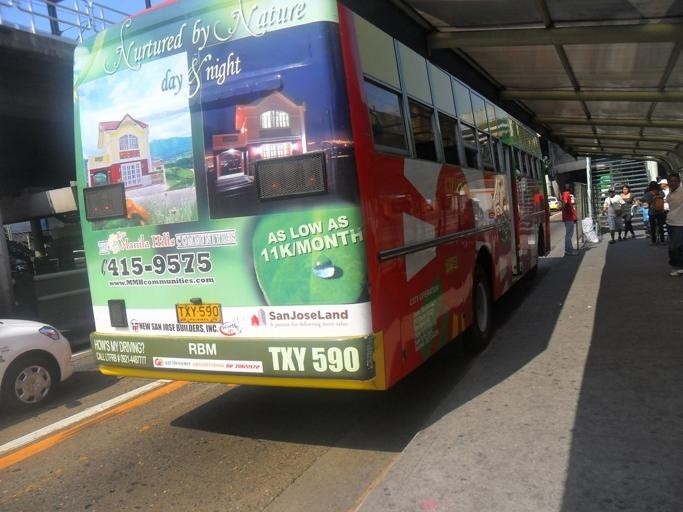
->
[72,4,551,416]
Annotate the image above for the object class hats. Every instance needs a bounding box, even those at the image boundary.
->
[658,179,668,184]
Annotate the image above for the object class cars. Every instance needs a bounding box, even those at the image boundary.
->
[548,197,563,211]
[73,249,86,268]
[570,194,576,204]
[0,316,75,414]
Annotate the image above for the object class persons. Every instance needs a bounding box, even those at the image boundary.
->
[639,180,665,245]
[657,178,669,242]
[637,201,649,237]
[559,183,579,256]
[601,189,625,244]
[619,184,636,239]
[664,173,682,276]
